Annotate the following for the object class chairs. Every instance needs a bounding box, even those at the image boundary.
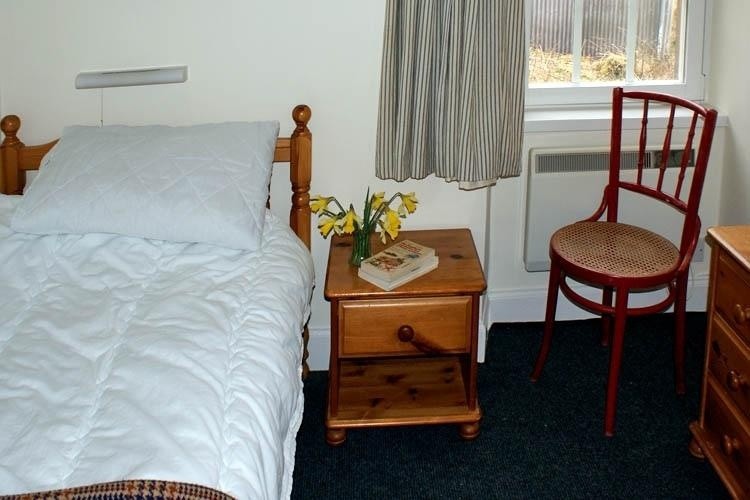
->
[537,89,718,435]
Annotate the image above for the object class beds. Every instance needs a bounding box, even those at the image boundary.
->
[0,101,317,499]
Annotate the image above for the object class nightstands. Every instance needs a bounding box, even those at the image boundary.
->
[323,222,488,443]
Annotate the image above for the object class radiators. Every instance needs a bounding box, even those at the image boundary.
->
[524,144,704,272]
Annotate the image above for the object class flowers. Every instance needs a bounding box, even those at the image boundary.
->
[309,186,421,245]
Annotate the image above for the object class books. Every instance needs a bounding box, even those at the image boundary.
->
[361,239,436,284]
[358,255,439,292]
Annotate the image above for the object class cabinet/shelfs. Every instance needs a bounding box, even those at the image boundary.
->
[688,224,750,499]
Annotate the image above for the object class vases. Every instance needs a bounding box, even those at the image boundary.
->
[350,231,372,267]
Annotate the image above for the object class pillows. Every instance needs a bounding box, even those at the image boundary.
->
[6,117,281,250]
